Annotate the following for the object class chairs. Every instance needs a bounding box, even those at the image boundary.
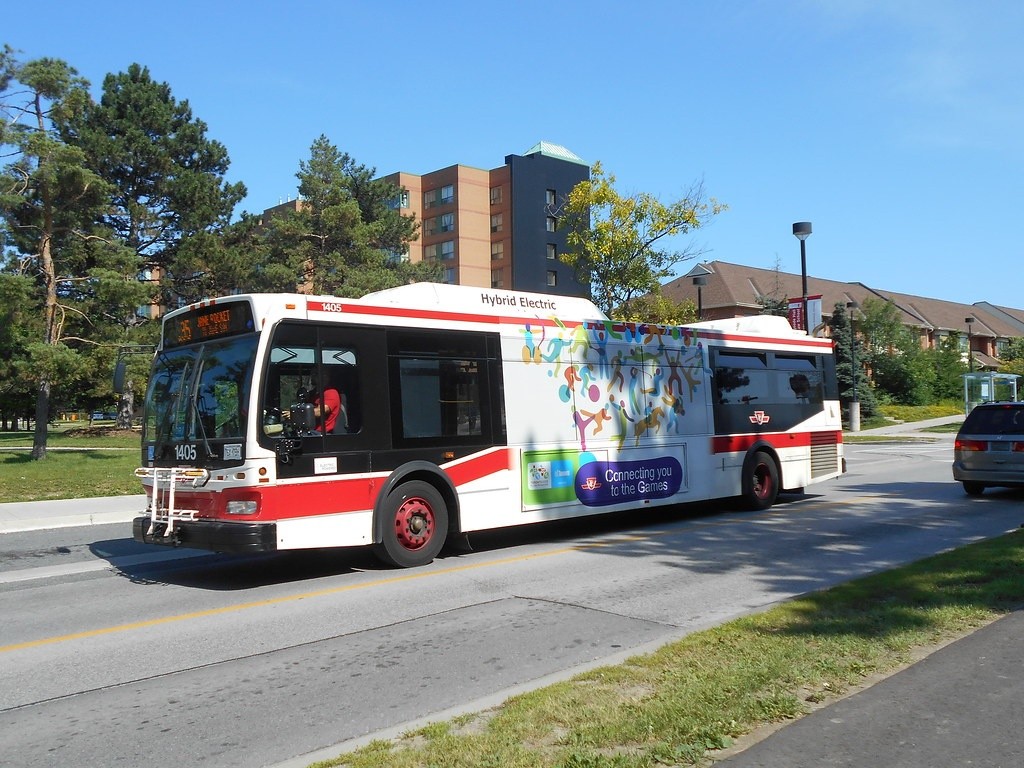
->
[330,373,353,433]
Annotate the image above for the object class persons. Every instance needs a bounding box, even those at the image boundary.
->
[281,365,340,436]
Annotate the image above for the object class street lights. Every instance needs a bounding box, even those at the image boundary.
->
[848,302,858,402]
[792,222,812,335]
[691,278,708,319]
[965,317,974,402]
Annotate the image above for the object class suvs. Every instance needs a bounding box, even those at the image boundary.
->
[951,399,1024,494]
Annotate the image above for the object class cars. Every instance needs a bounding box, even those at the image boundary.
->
[88,411,103,421]
[105,413,117,419]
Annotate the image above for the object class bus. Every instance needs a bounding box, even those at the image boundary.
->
[110,283,847,568]
